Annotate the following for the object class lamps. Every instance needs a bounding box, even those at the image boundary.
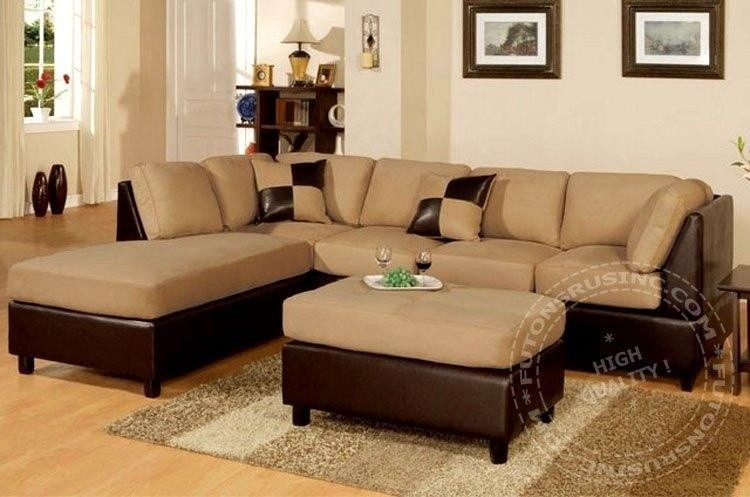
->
[281,19,317,87]
[362,13,380,68]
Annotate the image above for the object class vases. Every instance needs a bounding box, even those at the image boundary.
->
[30,107,51,120]
[32,173,49,217]
[48,165,67,214]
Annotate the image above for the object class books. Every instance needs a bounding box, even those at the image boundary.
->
[275,98,311,128]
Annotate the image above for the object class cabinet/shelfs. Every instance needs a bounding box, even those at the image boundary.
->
[236,84,344,162]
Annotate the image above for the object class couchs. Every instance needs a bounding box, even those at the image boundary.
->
[117,180,732,391]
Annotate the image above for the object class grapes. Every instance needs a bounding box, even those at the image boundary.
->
[382,267,417,287]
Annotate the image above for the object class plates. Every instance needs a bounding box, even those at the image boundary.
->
[362,272,444,291]
[237,93,258,117]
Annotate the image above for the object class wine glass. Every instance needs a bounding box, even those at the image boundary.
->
[373,240,392,285]
[415,246,434,286]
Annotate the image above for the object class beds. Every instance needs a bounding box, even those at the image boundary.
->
[6,230,312,398]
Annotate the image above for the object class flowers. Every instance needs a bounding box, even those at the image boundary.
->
[24,72,70,107]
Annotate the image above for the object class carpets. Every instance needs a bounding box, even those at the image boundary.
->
[103,351,750,497]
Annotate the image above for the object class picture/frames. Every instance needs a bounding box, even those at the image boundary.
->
[315,63,337,87]
[622,0,724,80]
[462,0,562,79]
